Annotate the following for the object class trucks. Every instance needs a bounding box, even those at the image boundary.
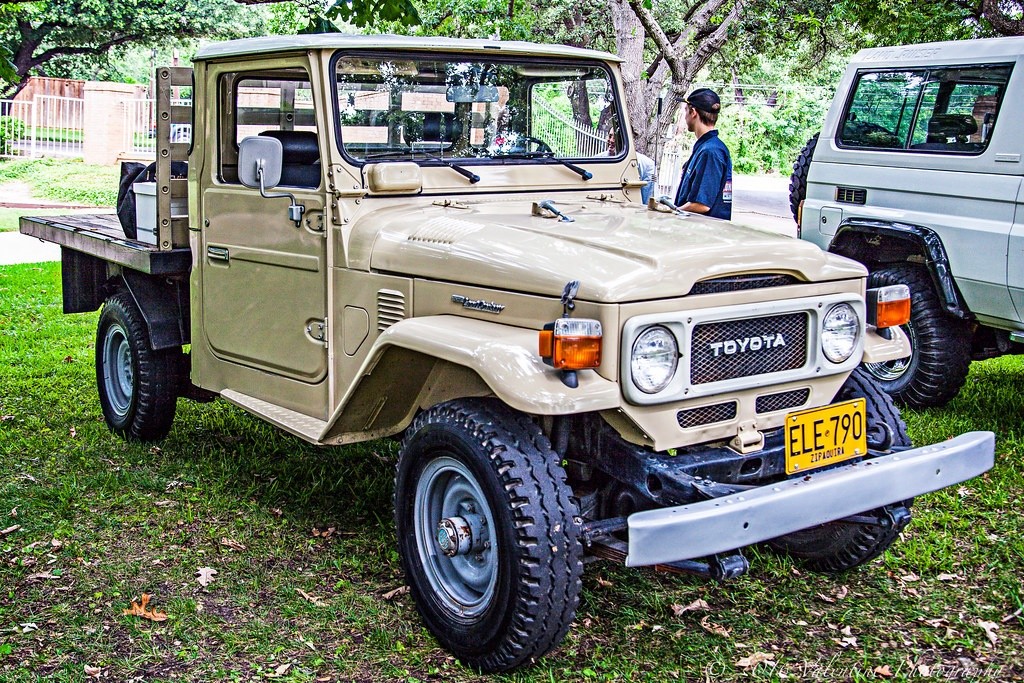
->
[13,32,999,675]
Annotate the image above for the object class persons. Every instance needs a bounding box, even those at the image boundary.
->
[674,88,733,221]
[591,123,655,205]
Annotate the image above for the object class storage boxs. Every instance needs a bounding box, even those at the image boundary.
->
[132,182,188,246]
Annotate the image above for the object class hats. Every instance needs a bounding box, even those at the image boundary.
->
[675,88,721,114]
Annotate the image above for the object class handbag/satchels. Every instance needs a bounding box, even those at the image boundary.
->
[116,157,188,239]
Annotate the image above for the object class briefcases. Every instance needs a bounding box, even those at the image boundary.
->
[133,179,190,245]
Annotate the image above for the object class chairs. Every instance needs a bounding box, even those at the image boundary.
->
[403,111,463,156]
[257,130,320,189]
[909,114,984,151]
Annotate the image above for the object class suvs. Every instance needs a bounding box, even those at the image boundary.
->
[788,35,1023,409]
